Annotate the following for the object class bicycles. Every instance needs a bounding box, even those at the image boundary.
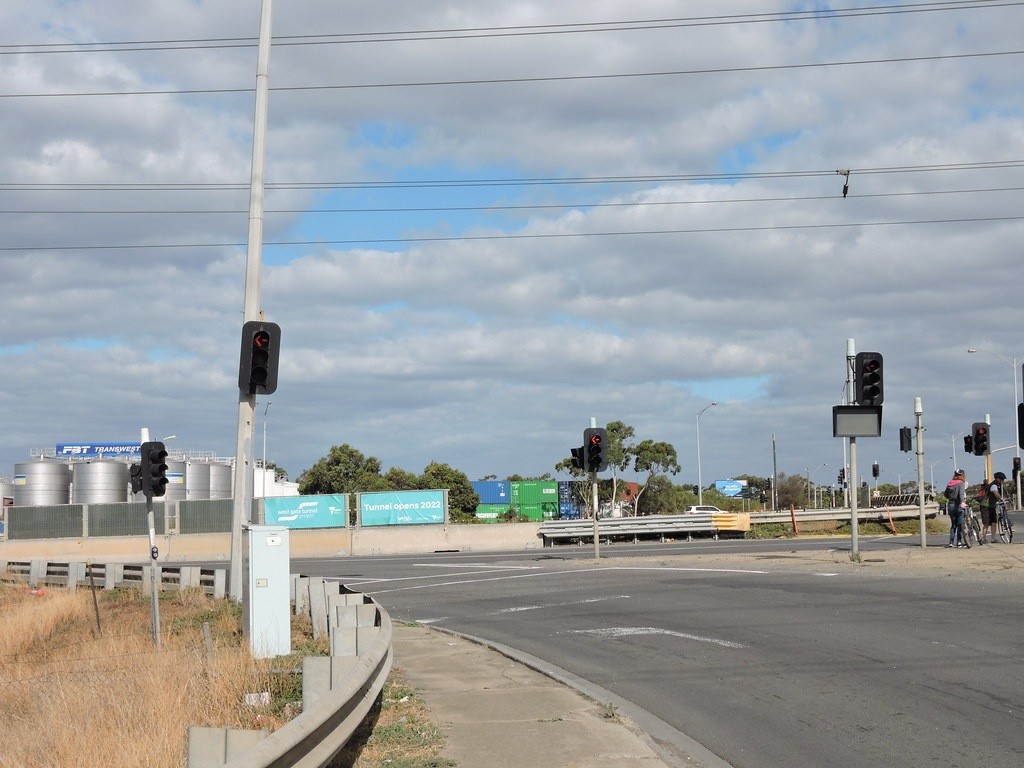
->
[961,498,982,548]
[995,498,1013,543]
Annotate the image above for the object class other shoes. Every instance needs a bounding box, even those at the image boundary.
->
[991,539,1001,543]
[982,539,989,544]
[949,541,967,548]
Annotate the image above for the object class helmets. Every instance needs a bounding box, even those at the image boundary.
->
[994,472,1007,480]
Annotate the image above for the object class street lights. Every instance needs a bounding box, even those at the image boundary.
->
[968,349,1022,511]
[804,463,827,488]
[696,402,717,506]
[263,401,272,525]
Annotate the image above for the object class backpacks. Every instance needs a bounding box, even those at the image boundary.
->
[944,482,963,501]
[974,482,999,503]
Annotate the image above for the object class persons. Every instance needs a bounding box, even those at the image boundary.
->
[980,472,1007,543]
[943,468,968,548]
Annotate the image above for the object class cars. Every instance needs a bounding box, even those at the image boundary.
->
[684,505,729,514]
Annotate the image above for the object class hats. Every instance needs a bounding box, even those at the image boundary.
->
[955,467,964,476]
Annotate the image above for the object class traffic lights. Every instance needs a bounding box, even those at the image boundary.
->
[872,464,879,477]
[765,478,770,489]
[570,446,584,470]
[855,352,884,405]
[765,497,767,502]
[964,435,972,452]
[972,422,990,455]
[838,468,845,485]
[238,321,281,395]
[693,485,698,495]
[1013,457,1021,471]
[584,428,607,472]
[760,495,764,503]
[141,442,169,497]
